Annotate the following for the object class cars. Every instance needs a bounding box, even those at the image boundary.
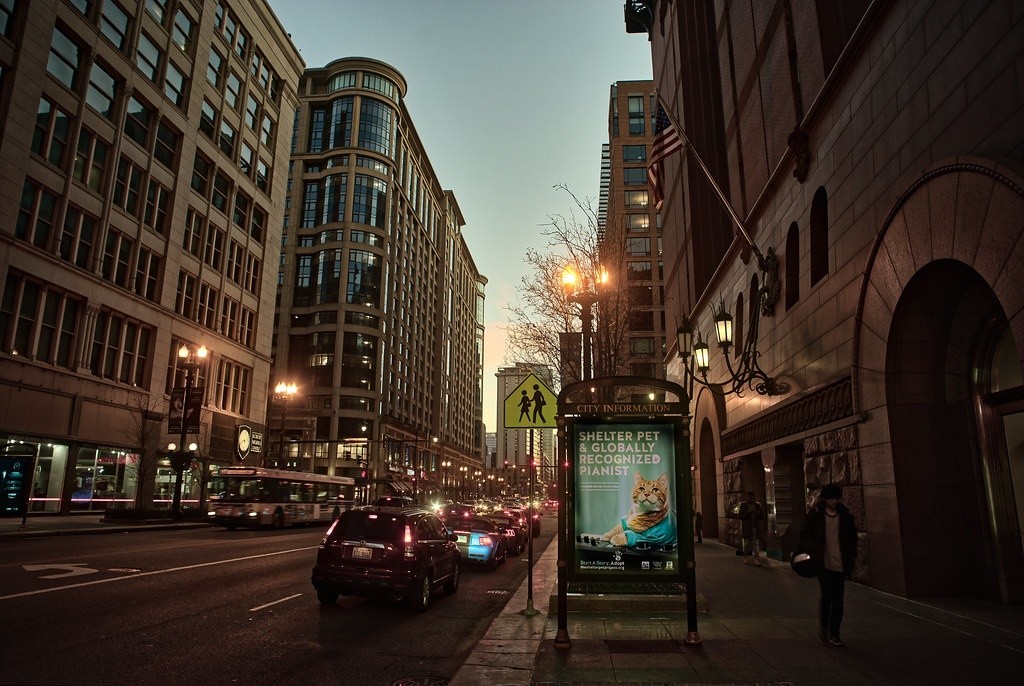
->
[351,495,558,572]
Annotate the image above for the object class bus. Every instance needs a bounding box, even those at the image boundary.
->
[205,466,355,532]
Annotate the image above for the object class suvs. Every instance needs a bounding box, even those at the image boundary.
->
[311,506,461,614]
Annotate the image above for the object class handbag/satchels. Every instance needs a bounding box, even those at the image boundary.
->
[790,506,823,578]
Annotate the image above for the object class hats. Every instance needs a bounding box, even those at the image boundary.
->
[818,484,843,499]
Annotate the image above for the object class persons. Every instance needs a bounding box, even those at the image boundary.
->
[695,512,703,544]
[739,492,763,563]
[803,483,857,647]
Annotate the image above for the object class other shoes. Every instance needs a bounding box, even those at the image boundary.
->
[816,628,830,646]
[828,634,845,646]
[753,558,762,567]
[742,556,750,564]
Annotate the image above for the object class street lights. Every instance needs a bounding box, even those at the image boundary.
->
[563,265,609,405]
[167,343,208,519]
[274,380,297,470]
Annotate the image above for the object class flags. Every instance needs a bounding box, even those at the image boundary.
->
[647,102,681,209]
